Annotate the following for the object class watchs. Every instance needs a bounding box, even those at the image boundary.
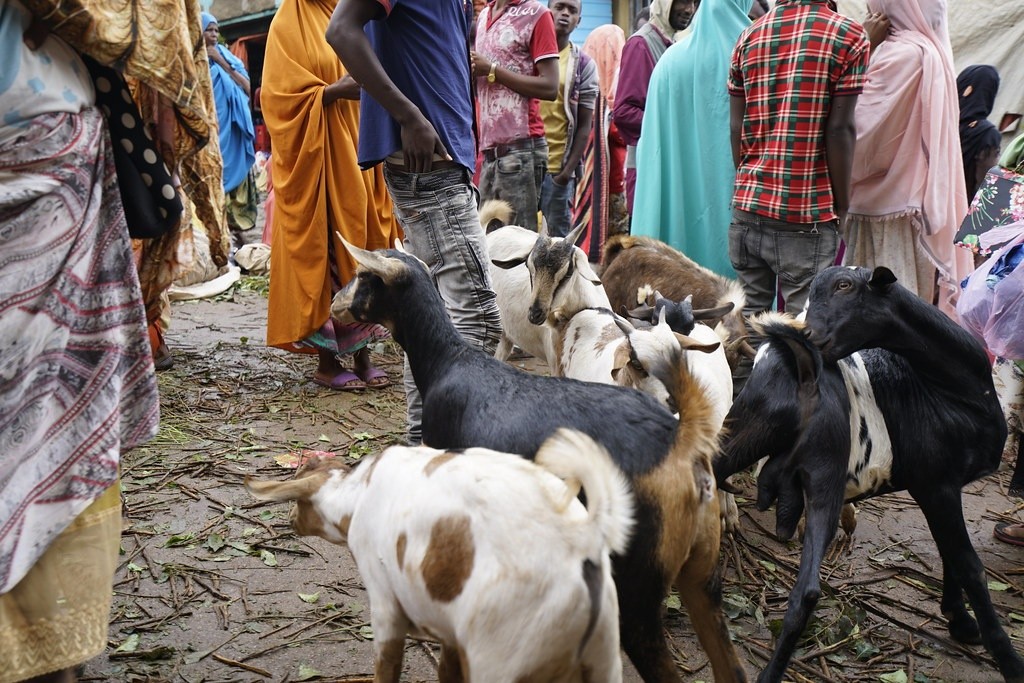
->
[488,63,496,83]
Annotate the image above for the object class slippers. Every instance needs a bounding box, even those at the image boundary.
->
[360,369,392,387]
[313,371,367,390]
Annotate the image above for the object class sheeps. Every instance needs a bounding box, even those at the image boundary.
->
[244,200,1024,683]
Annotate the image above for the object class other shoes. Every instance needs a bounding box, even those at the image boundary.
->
[993,523,1024,545]
[155,342,173,370]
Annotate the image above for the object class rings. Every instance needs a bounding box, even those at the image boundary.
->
[873,14,878,17]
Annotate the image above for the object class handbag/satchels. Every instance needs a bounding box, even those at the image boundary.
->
[78,50,183,238]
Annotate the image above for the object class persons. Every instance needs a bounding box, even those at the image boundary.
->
[202,13,257,246]
[322,0,503,447]
[259,0,403,390]
[0,0,217,683]
[468,0,1024,545]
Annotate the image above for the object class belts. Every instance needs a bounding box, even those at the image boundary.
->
[535,136,547,148]
[487,139,534,162]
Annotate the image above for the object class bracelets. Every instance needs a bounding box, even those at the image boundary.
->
[230,65,236,72]
